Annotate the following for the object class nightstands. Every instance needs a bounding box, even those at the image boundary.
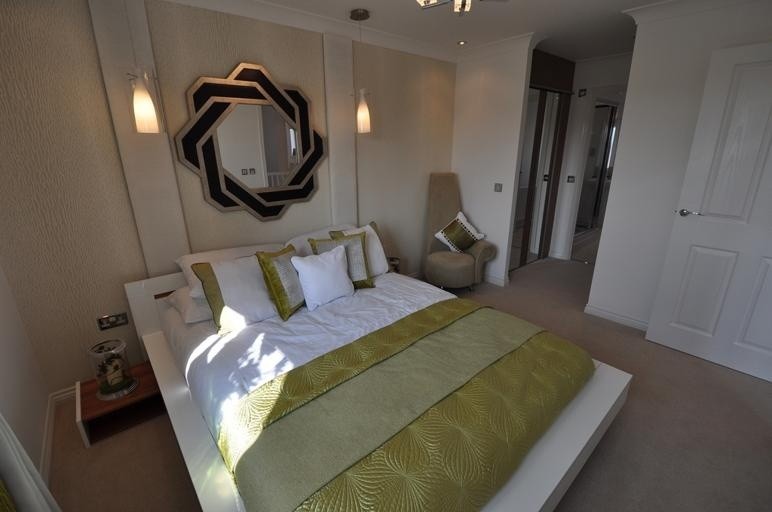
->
[76,360,168,449]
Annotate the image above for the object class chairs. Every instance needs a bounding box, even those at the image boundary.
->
[423,172,497,291]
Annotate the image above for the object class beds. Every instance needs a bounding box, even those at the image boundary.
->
[124,272,634,512]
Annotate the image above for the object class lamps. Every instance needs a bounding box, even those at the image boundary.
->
[127,68,159,134]
[417,0,473,17]
[351,89,371,134]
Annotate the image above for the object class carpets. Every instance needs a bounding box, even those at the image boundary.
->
[572,233,600,263]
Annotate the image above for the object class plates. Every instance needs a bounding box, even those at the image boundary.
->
[95,378,140,400]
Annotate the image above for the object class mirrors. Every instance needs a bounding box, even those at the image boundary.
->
[174,61,328,221]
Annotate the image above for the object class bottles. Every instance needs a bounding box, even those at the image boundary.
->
[88,338,132,392]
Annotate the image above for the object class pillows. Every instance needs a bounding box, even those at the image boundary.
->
[433,211,486,254]
[167,221,394,333]
